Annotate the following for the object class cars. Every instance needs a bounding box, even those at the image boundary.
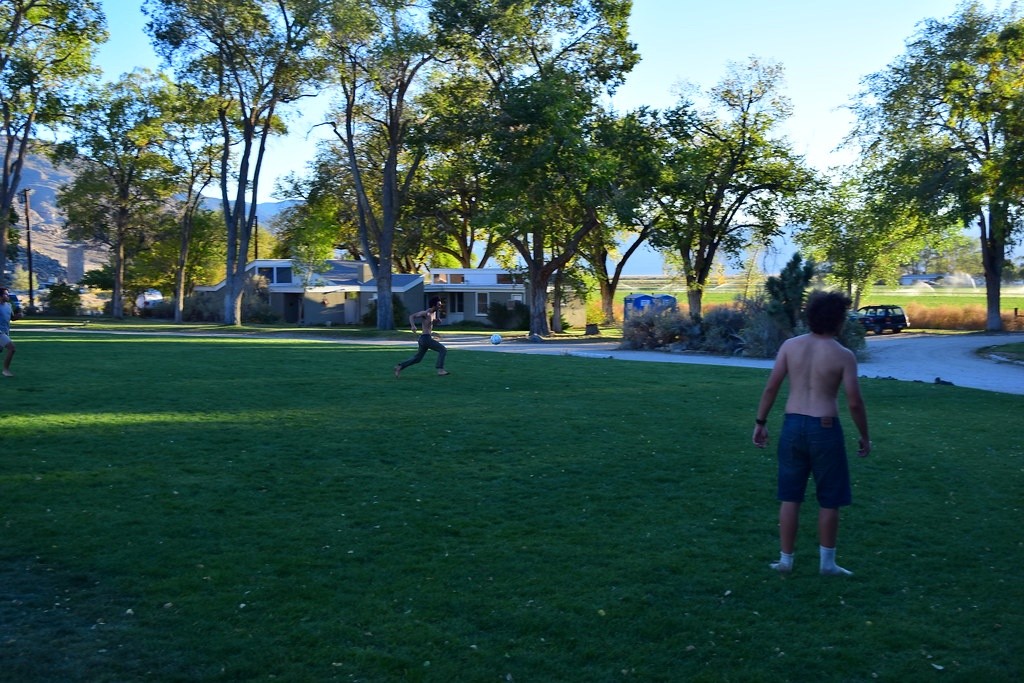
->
[848,303,910,336]
[6,292,23,313]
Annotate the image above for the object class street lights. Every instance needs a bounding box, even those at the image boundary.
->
[16,188,36,307]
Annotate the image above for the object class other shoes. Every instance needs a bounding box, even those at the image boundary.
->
[820,565,854,576]
[2,372,13,376]
[439,370,450,375]
[770,562,792,572]
[393,366,399,377]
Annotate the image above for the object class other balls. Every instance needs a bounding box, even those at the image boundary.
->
[490,334,502,344]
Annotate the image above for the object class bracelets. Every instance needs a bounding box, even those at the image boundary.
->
[756,419,766,425]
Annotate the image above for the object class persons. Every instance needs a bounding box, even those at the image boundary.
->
[395,296,450,377]
[752,289,870,576]
[0,288,16,376]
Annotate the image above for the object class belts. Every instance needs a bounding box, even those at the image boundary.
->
[421,334,431,336]
